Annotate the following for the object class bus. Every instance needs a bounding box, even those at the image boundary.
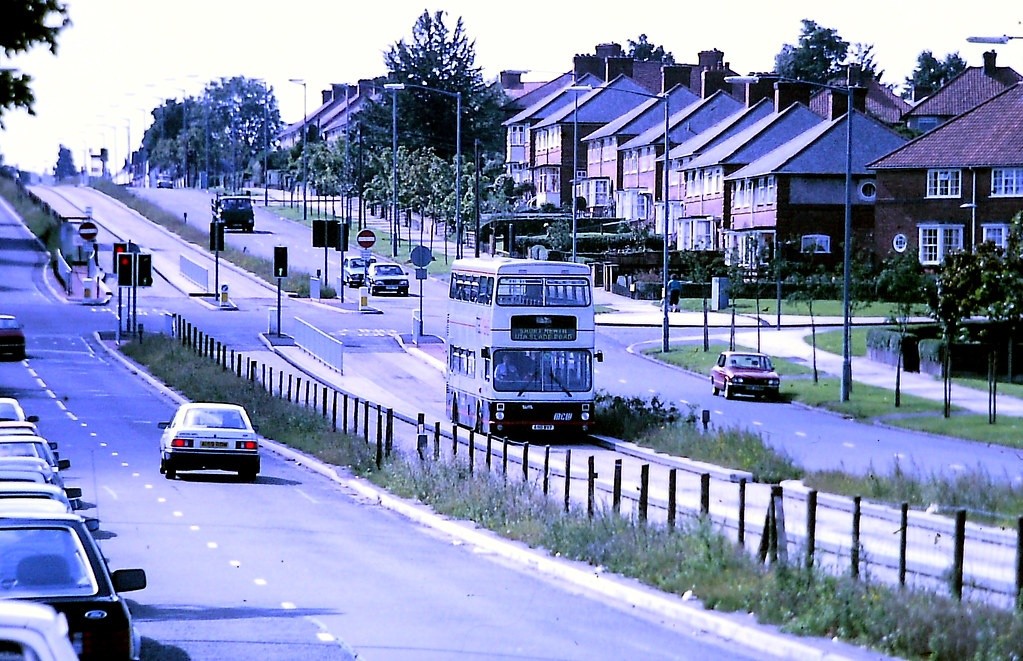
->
[444,257,604,438]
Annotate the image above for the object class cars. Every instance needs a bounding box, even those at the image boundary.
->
[157,401,260,482]
[0,395,148,661]
[710,351,780,400]
[157,174,174,189]
[365,262,409,296]
[0,314,26,357]
[343,256,377,288]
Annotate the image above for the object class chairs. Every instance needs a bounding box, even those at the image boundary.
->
[221,419,240,428]
[15,554,71,585]
[751,361,758,367]
[731,359,737,364]
[389,269,396,275]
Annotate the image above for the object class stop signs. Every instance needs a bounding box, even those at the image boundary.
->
[357,229,377,248]
[78,222,99,239]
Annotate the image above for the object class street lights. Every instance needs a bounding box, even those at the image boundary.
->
[507,69,578,263]
[724,75,853,402]
[288,78,308,220]
[564,85,670,353]
[383,83,460,259]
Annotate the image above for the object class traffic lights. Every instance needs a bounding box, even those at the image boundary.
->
[117,252,133,287]
[137,253,153,286]
[113,242,128,273]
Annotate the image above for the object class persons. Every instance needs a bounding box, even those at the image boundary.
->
[543,223,555,237]
[495,354,518,381]
[668,274,680,312]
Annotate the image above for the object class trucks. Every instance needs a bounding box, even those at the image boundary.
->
[211,191,255,233]
